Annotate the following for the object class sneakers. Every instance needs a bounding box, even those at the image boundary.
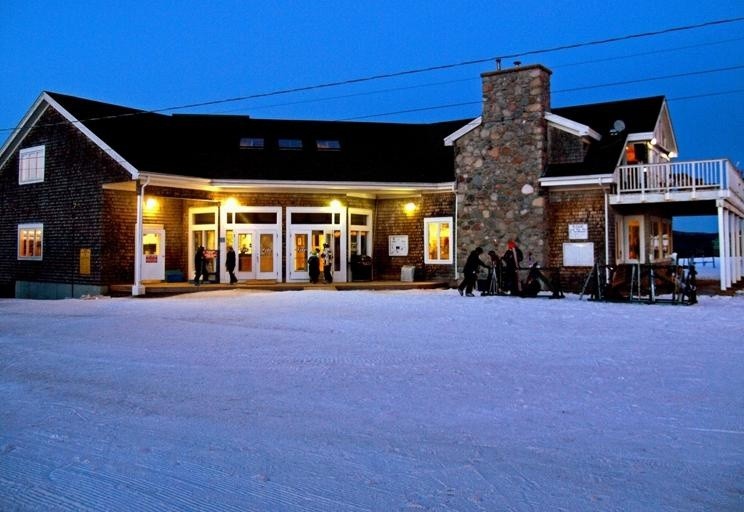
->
[466,293,474,296]
[458,288,463,296]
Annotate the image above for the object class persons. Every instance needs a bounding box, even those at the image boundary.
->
[226,246,237,285]
[194,246,210,286]
[307,243,333,284]
[457,242,523,296]
